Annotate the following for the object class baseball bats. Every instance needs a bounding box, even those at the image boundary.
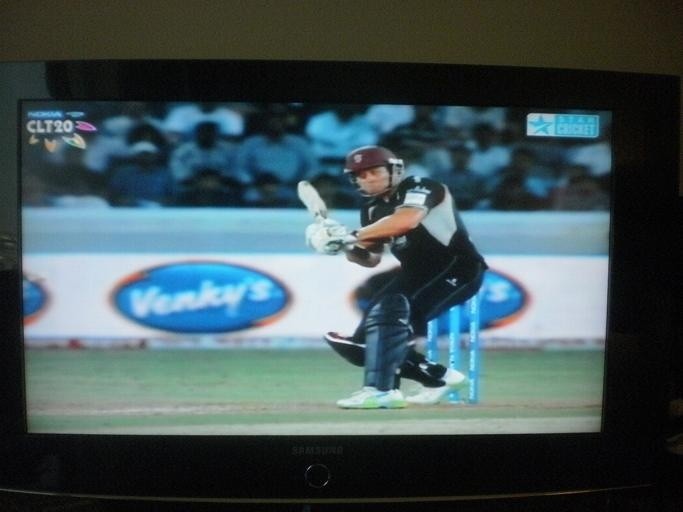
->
[298,180,341,252]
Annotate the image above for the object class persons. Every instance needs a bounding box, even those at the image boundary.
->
[21,101,611,210]
[322,145,488,411]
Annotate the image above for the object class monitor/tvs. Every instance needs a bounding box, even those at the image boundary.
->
[0,58,683,512]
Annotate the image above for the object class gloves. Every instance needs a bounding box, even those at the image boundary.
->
[305,215,356,254]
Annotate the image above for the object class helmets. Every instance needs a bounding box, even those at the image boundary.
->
[344,145,403,175]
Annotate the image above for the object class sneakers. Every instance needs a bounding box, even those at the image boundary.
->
[406,368,464,406]
[336,386,402,409]
